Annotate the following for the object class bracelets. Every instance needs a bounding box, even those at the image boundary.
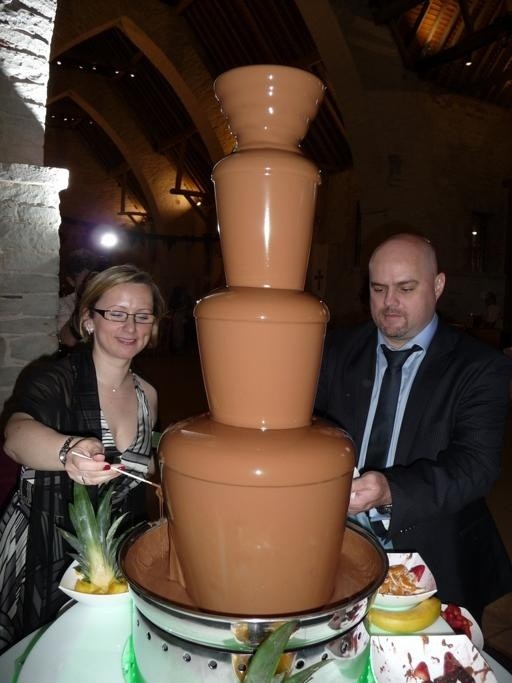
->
[378,504,392,513]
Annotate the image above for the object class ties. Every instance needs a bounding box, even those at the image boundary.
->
[364,344,424,505]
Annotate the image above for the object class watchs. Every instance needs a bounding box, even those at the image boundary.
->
[60,435,75,465]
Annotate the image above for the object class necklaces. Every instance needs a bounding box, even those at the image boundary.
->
[111,383,120,391]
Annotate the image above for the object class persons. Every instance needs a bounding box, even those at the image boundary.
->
[60,270,99,345]
[313,231,510,631]
[1,263,166,653]
[57,246,111,327]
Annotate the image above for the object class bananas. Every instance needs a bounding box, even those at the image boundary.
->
[368,596,441,633]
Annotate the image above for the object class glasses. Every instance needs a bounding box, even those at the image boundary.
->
[87,306,155,327]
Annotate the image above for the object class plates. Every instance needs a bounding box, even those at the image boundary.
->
[370,551,438,612]
[367,604,498,683]
[57,553,131,607]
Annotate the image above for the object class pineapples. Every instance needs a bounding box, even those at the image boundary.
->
[57,476,132,594]
